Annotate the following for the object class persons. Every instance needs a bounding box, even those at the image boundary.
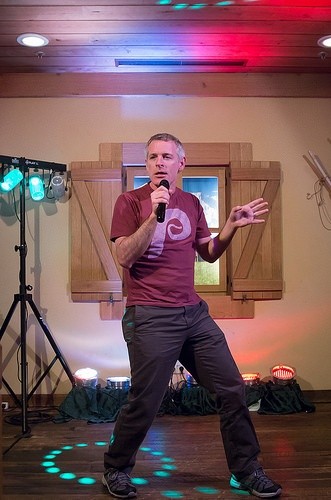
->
[101,134,282,499]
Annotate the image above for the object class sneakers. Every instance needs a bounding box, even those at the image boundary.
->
[229,465,283,498]
[101,467,138,498]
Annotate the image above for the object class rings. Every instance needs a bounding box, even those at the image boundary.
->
[254,212,256,217]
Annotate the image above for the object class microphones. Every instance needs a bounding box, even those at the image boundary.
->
[156,180,169,223]
